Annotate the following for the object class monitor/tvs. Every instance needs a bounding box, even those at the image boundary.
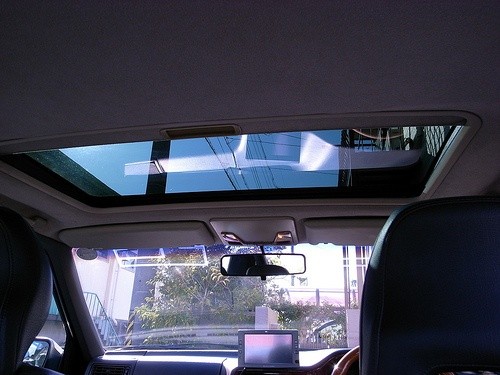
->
[238,328,300,368]
